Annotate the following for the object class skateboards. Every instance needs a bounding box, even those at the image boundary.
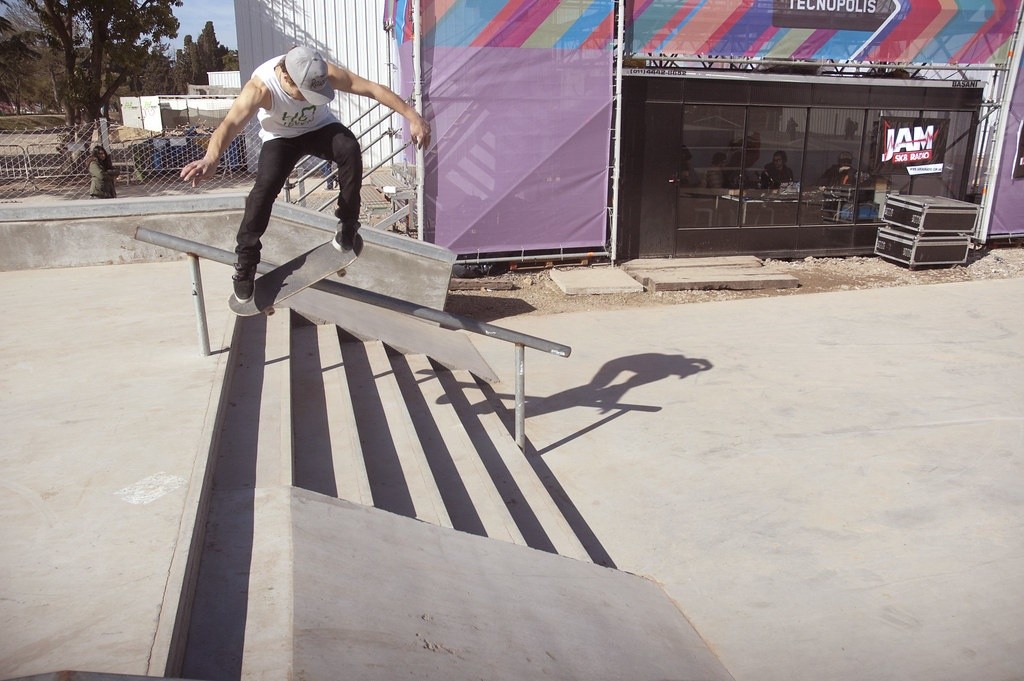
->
[226,232,365,319]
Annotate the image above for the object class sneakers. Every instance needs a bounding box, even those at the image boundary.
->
[232,262,257,304]
[332,220,361,254]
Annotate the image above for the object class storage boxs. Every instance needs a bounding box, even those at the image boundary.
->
[821,186,891,222]
[873,224,971,271]
[881,193,981,234]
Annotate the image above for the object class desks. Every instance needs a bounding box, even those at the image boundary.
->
[715,192,824,228]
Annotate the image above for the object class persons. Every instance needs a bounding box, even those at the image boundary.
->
[819,151,870,208]
[180,46,431,304]
[680,144,743,226]
[786,116,798,140]
[753,149,794,248]
[86,146,119,197]
[845,117,856,139]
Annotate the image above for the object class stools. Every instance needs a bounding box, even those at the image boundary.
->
[695,208,719,227]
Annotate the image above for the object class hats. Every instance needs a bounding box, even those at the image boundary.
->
[285,47,335,106]
